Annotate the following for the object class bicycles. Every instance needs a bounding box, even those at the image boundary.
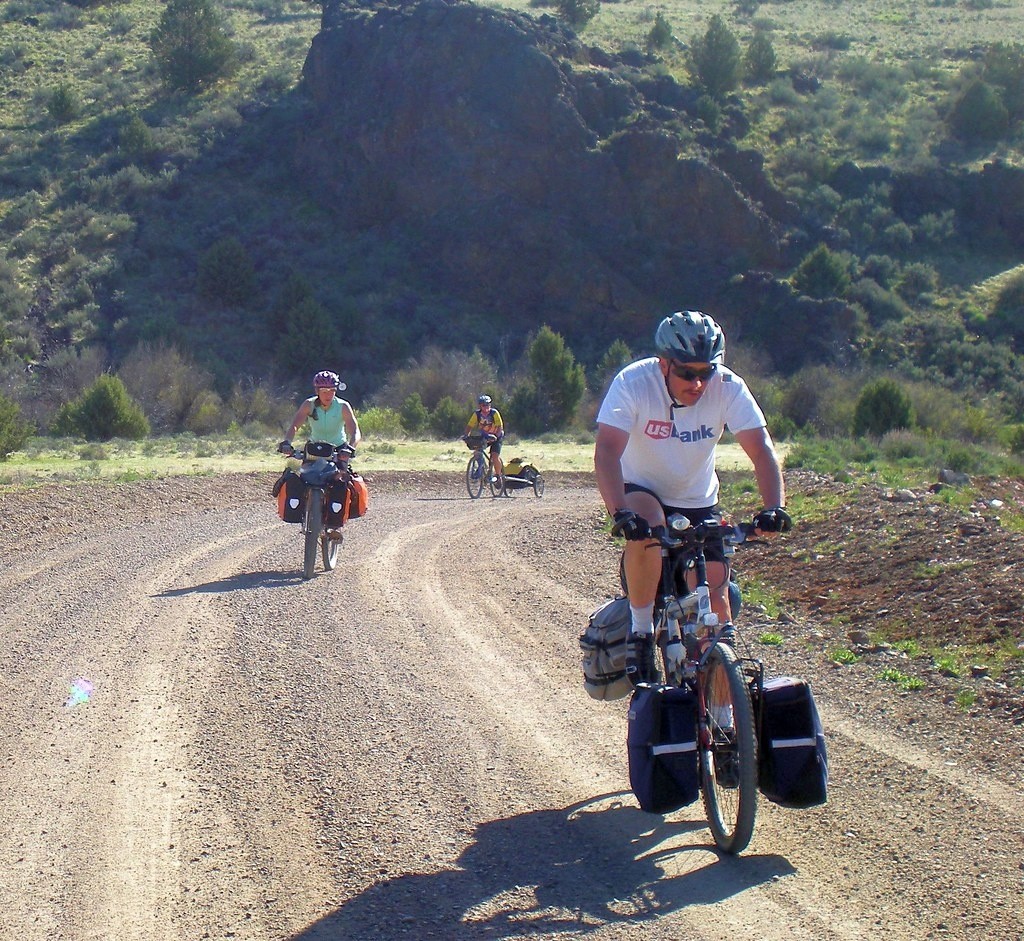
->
[463,435,505,498]
[623,522,765,853]
[281,442,352,580]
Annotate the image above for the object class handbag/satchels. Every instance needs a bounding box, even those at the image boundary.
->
[348,474,368,519]
[279,473,306,523]
[326,484,349,527]
[753,677,827,809]
[579,596,635,701]
[628,682,699,813]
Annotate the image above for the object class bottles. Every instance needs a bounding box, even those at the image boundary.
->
[665,635,686,682]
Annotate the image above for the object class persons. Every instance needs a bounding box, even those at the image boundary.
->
[464,396,504,483]
[594,310,786,754]
[279,371,361,543]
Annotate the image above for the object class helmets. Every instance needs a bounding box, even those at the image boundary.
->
[654,310,725,364]
[479,395,491,403]
[313,371,339,386]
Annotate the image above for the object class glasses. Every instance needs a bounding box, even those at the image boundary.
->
[670,360,718,381]
[319,387,337,392]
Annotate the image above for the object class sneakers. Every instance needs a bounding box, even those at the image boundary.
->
[713,726,740,788]
[626,623,655,689]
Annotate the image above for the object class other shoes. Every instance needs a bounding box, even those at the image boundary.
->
[325,526,343,545]
[493,475,501,489]
[475,466,482,477]
